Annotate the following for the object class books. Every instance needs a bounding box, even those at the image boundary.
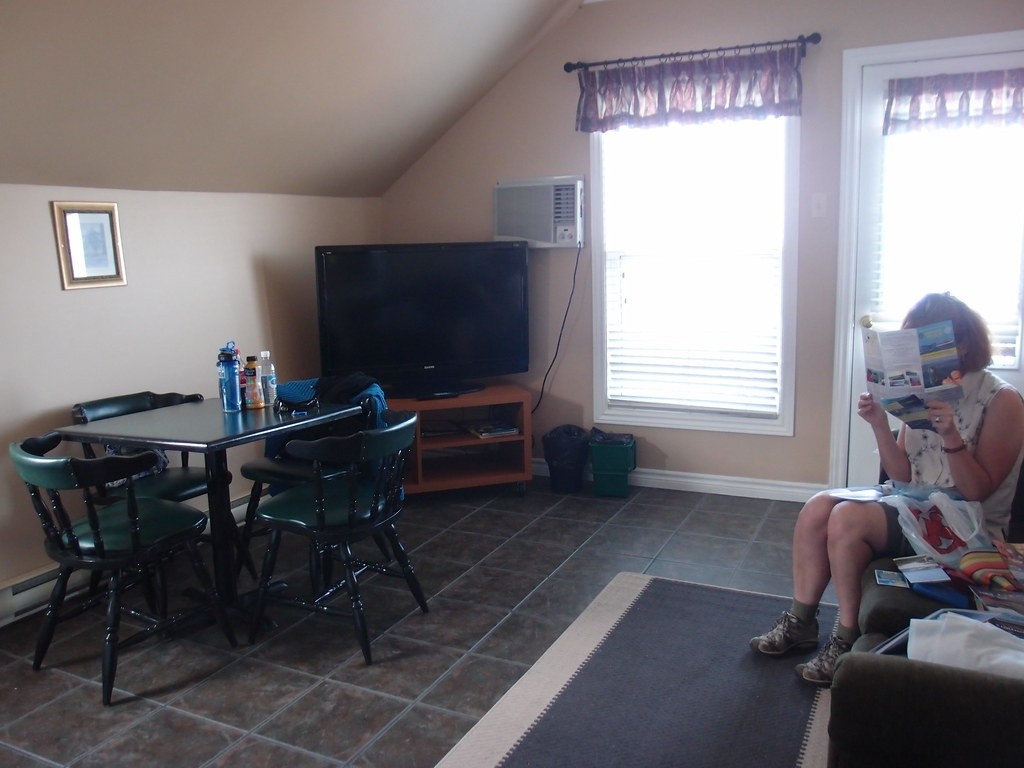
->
[969,584,1024,636]
[461,418,520,439]
[421,420,462,437]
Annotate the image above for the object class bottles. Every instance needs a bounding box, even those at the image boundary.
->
[244,356,265,409]
[258,350,278,406]
[216,352,243,412]
[231,348,247,400]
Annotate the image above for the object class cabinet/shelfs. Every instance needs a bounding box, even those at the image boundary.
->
[383,379,533,497]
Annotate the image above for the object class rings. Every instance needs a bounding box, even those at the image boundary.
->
[936,416,940,422]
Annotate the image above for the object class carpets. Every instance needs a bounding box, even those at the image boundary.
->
[432,571,840,768]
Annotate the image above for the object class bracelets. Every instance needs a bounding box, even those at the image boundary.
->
[941,436,968,453]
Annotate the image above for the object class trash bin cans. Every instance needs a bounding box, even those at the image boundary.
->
[587,434,636,497]
[544,424,588,493]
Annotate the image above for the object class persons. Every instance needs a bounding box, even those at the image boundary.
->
[928,365,938,386]
[749,290,1024,684]
[951,368,961,383]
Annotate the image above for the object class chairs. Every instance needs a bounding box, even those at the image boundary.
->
[7,372,430,708]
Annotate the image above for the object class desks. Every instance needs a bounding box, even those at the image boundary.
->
[53,398,363,641]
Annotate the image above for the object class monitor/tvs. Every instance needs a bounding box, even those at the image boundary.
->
[315,240,529,398]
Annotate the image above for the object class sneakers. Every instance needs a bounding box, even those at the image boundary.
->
[795,635,852,688]
[748,610,819,655]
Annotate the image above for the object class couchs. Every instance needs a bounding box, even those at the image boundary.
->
[827,429,1024,768]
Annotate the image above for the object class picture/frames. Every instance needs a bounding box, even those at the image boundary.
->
[52,201,128,291]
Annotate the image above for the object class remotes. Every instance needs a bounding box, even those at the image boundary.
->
[418,391,459,400]
